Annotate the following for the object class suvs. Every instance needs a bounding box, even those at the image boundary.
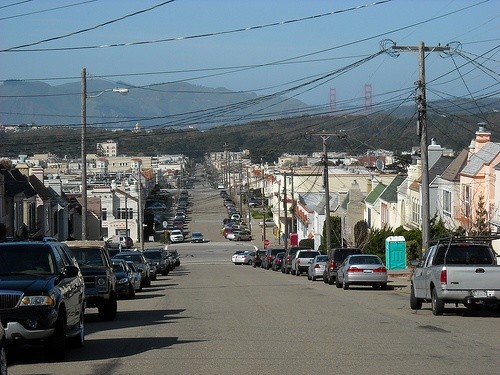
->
[291,249,321,276]
[253,249,267,268]
[261,249,286,270]
[281,247,311,274]
[323,248,363,285]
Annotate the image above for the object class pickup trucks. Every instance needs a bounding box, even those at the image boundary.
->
[409,239,500,317]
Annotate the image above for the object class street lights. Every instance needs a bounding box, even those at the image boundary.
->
[82,70,129,267]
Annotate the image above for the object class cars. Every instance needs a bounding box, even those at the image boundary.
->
[335,254,388,290]
[231,250,255,265]
[258,218,275,228]
[271,252,286,272]
[0,236,181,375]
[169,189,189,244]
[307,255,327,281]
[190,232,204,243]
[220,190,252,241]
